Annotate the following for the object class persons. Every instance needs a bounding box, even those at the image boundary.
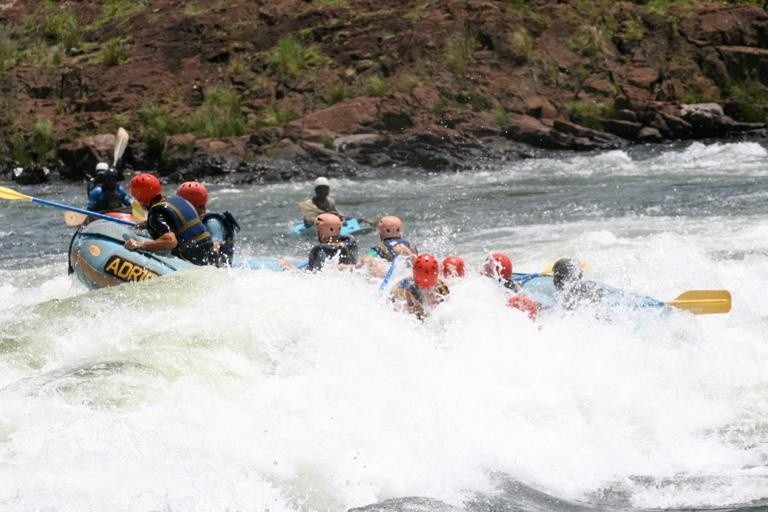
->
[308,213,359,271]
[87,161,133,212]
[552,257,603,310]
[390,253,450,322]
[304,177,336,228]
[124,173,215,267]
[362,216,418,277]
[484,253,517,293]
[177,182,241,268]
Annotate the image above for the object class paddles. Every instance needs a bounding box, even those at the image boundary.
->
[609,290,732,314]
[513,257,590,281]
[111,126,129,170]
[0,186,148,230]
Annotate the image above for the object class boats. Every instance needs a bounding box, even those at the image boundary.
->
[69,212,664,317]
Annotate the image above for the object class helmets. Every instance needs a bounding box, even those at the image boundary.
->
[379,216,403,240]
[176,181,208,208]
[486,253,512,281]
[413,253,439,287]
[315,213,342,239]
[553,256,582,279]
[128,173,163,206]
[505,294,536,319]
[315,176,330,189]
